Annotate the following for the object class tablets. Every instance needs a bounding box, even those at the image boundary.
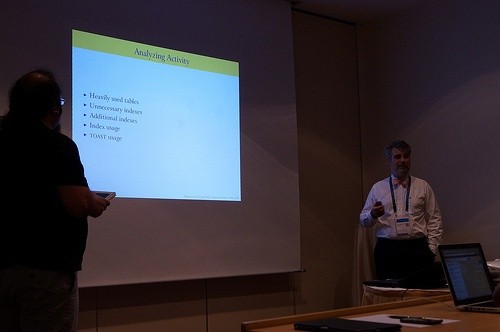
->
[96,192,115,201]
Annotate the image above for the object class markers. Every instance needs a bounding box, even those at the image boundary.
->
[400,318,444,325]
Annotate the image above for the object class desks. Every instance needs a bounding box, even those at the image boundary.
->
[241,294,500,332]
[362,284,450,306]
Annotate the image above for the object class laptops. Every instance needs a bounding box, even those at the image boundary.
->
[437,243,500,313]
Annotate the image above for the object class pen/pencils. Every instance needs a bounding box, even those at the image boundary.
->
[388,315,424,319]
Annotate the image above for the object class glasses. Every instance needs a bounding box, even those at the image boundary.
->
[52,97,65,105]
[391,152,409,160]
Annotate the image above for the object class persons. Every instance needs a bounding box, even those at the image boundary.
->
[0,68,111,332]
[359,141,445,288]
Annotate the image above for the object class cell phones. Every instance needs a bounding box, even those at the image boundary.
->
[400,318,443,324]
